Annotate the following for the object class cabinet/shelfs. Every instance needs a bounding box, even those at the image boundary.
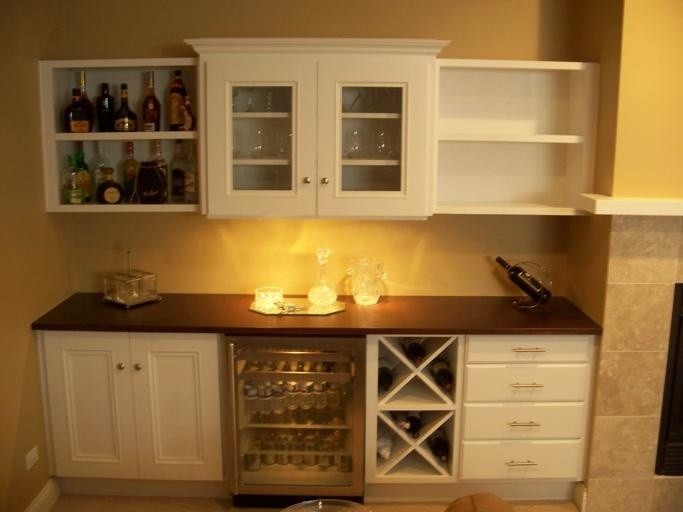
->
[33,325,226,498]
[226,327,364,498]
[36,58,202,213]
[437,58,601,218]
[363,329,459,504]
[178,36,452,221]
[459,332,601,503]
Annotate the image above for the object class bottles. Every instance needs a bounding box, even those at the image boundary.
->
[62,69,196,205]
[378,341,453,462]
[307,250,337,306]
[232,88,291,158]
[243,360,351,471]
[495,256,551,304]
[348,126,392,159]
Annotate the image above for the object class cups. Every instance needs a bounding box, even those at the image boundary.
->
[345,258,384,306]
[256,287,283,309]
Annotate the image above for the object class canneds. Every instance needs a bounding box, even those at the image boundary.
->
[244,402,352,473]
